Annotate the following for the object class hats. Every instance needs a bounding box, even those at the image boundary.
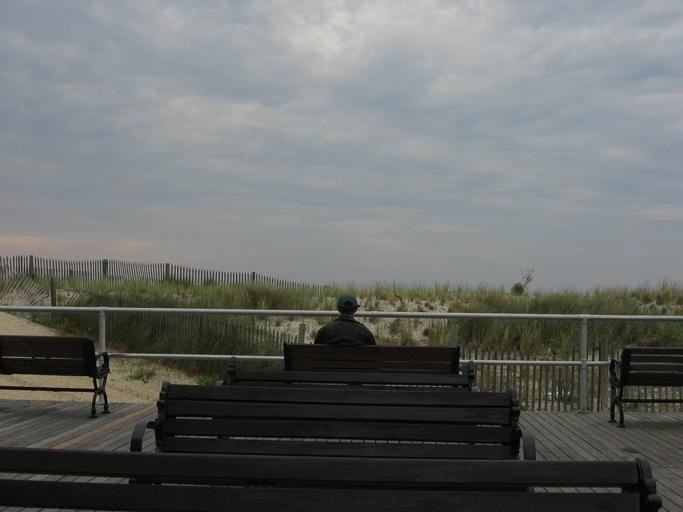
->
[338,294,360,309]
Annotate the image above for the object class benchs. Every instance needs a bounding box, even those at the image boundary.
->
[0,444,665,511]
[608,347,683,428]
[0,334,110,417]
[220,341,477,393]
[130,379,537,460]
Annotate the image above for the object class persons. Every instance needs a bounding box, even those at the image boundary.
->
[314,293,376,388]
